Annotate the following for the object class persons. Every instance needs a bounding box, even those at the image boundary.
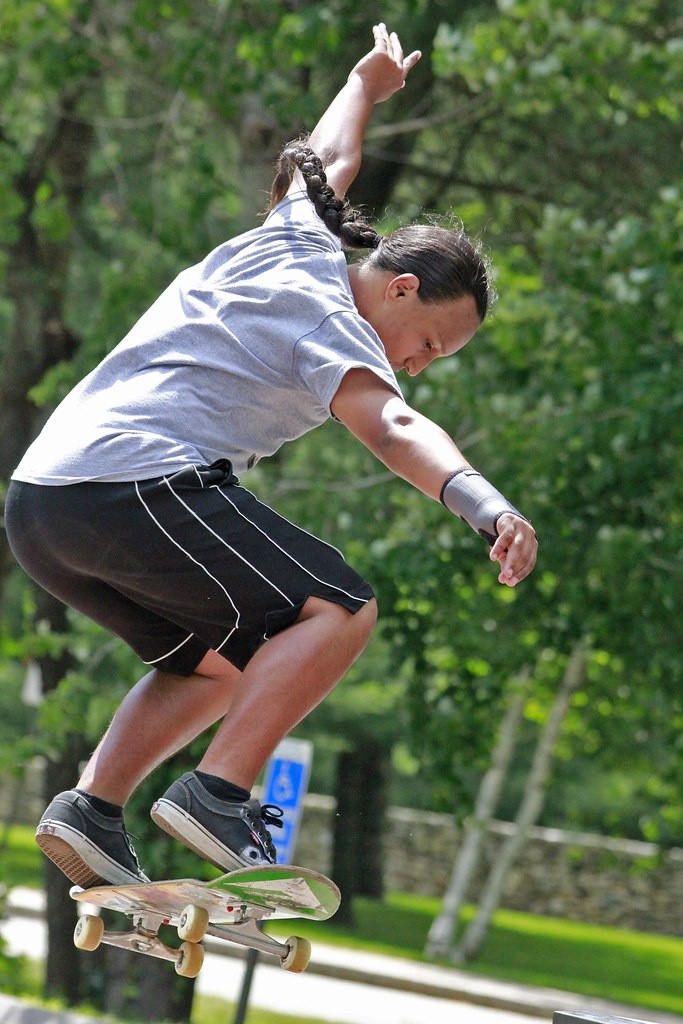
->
[2,19,541,894]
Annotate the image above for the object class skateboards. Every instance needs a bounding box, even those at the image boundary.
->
[68,863,343,979]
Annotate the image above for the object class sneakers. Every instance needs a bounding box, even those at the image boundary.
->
[35,790,151,889]
[150,772,283,874]
